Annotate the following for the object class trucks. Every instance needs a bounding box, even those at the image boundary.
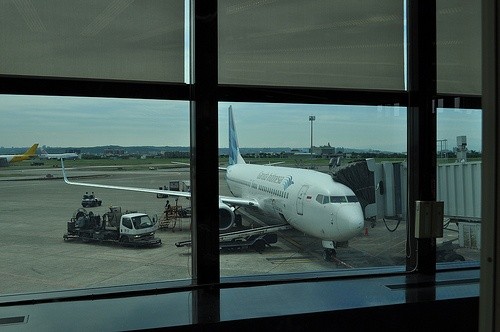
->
[62,205,162,247]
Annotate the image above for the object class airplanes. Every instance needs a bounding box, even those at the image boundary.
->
[39,146,78,161]
[60,104,365,261]
[0,143,39,167]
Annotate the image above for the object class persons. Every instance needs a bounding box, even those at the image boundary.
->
[83,191,90,199]
[89,191,95,199]
[164,200,171,219]
